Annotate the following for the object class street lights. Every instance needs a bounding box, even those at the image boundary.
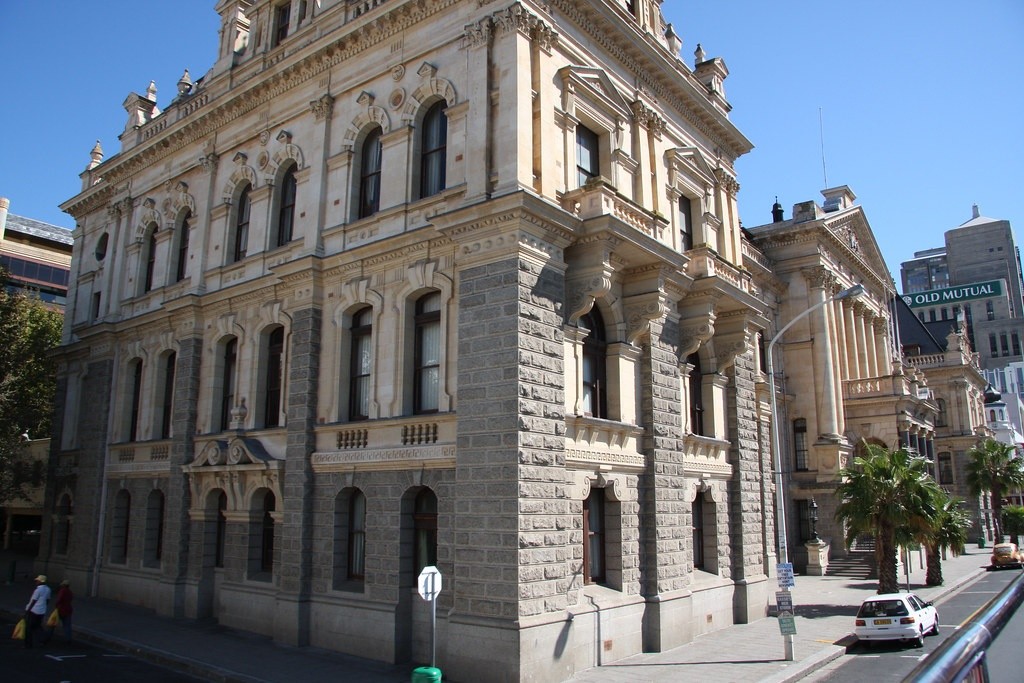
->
[767,284,865,661]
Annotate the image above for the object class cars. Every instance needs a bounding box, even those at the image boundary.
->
[989,543,1024,569]
[854,592,941,649]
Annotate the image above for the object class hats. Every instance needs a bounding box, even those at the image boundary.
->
[34,575,48,582]
[60,580,70,585]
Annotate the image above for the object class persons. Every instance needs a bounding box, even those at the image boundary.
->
[53,579,74,644]
[21,574,52,652]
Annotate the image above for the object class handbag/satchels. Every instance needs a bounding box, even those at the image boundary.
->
[46,609,60,627]
[11,618,26,640]
